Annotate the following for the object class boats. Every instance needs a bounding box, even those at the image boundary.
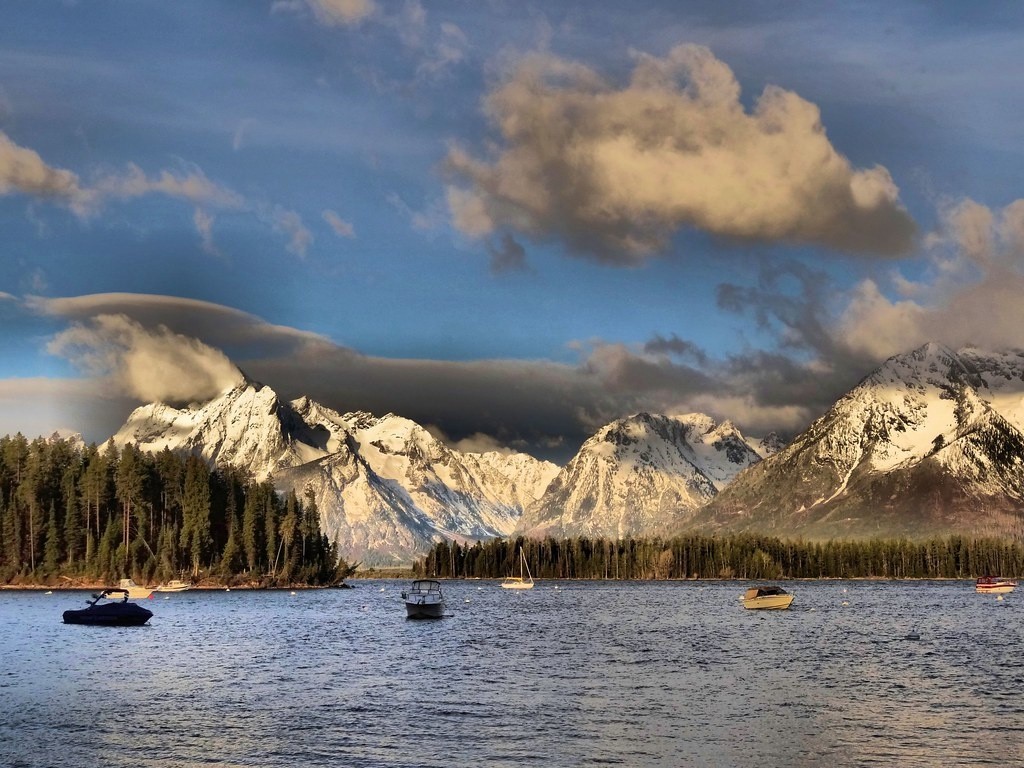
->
[149,580,189,592]
[63,586,154,628]
[100,579,155,600]
[976,575,1016,595]
[743,585,794,611]
[398,580,446,617]
[502,546,534,590]
